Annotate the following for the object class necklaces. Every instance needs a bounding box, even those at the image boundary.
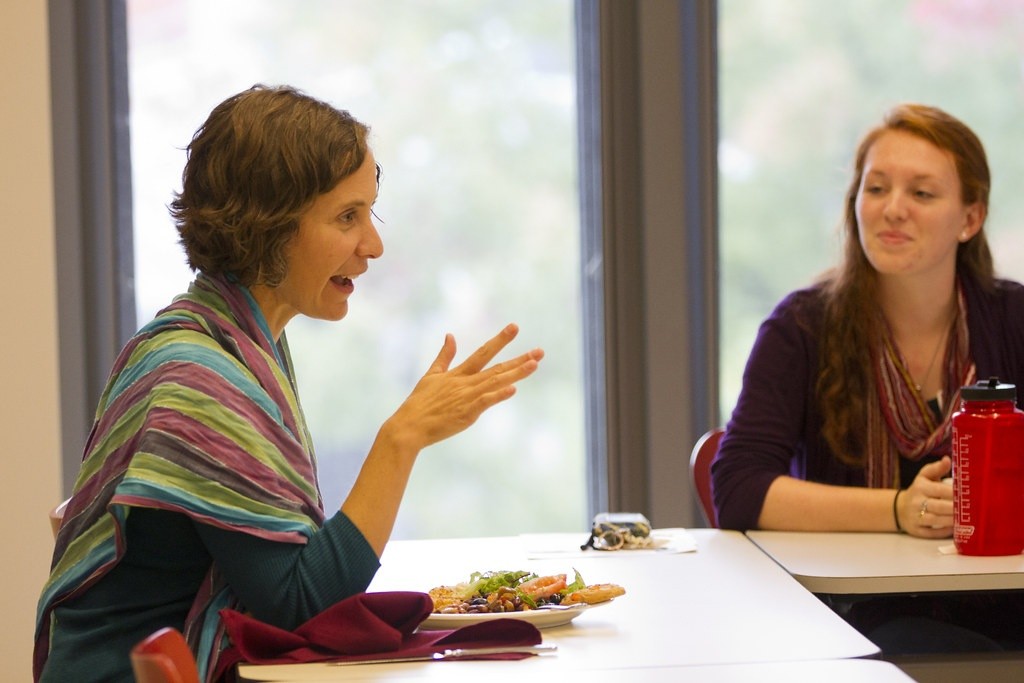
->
[913,297,956,392]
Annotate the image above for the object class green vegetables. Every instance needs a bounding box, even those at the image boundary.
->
[463,568,585,608]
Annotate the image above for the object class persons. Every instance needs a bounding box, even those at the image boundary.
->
[710,102,1023,540]
[30,82,546,683]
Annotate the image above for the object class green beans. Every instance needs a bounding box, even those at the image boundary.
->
[450,597,524,613]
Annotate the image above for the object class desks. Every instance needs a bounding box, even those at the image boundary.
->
[237,527,916,682]
[744,525,1023,595]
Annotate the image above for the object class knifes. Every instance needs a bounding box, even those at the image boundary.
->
[334,645,557,665]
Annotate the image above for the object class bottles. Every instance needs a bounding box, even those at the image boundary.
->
[954,374,1024,557]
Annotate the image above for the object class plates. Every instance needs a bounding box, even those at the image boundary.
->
[419,608,585,632]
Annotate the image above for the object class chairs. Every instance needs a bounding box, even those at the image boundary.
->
[50,496,74,537]
[689,428,728,529]
[129,625,200,683]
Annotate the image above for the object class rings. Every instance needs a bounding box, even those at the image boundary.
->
[923,498,929,513]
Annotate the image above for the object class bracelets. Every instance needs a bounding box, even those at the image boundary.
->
[893,487,904,534]
